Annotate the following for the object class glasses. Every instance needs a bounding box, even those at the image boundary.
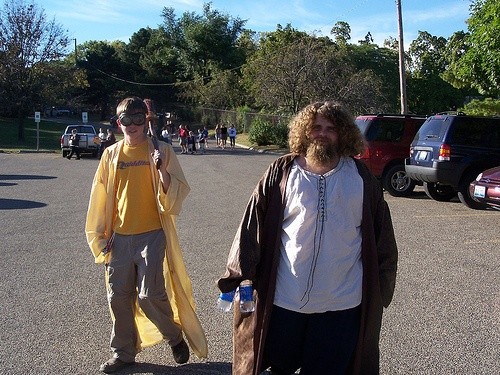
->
[120,112,146,126]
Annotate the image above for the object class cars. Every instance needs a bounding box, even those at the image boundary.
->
[60,124,101,158]
[104,114,118,126]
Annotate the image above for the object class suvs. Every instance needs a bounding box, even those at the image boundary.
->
[351,113,432,197]
[405,111,500,210]
[45,106,74,117]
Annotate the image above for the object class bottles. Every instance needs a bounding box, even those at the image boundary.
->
[216,287,236,312]
[238,279,255,313]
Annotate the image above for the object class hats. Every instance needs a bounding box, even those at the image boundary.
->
[107,128,112,132]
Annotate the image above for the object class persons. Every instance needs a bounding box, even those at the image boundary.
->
[215,101,398,375]
[94,128,116,159]
[66,129,81,160]
[160,110,209,154]
[215,123,227,150]
[229,124,236,149]
[85,97,191,372]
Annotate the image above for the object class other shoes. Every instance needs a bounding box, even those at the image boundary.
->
[75,158,80,160]
[100,358,135,374]
[66,156,70,160]
[171,338,189,364]
